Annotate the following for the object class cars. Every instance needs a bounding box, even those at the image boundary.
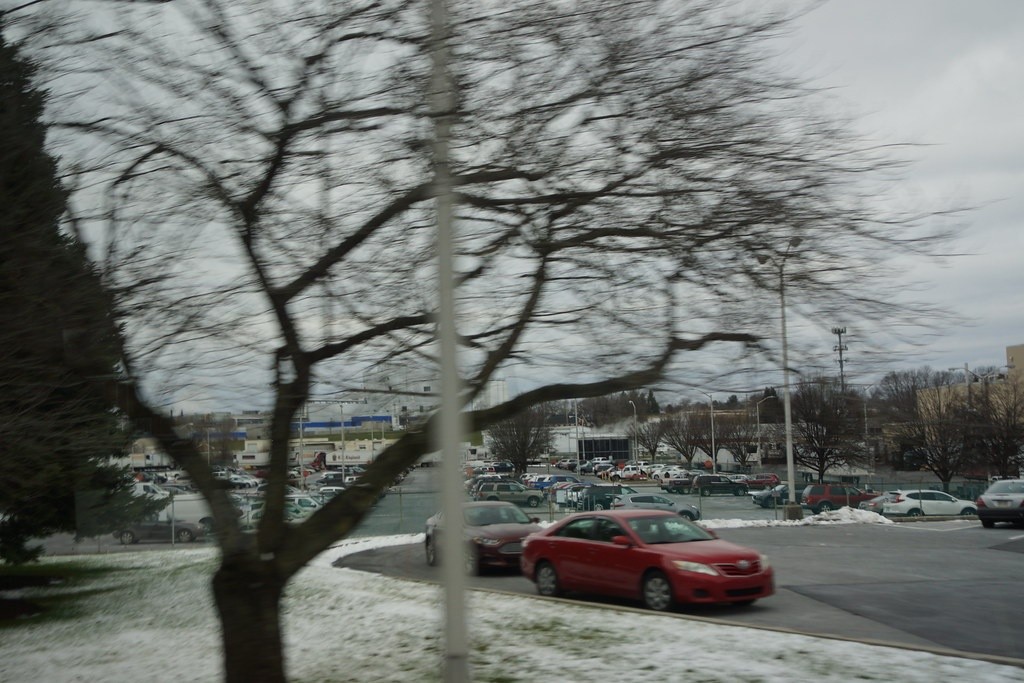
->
[859,496,886,515]
[460,456,778,506]
[111,440,445,546]
[425,500,545,576]
[518,510,775,612]
[610,493,702,522]
[979,479,1024,528]
[882,488,979,517]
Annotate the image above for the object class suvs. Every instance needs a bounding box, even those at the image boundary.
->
[800,481,879,515]
[752,481,807,509]
[473,480,544,508]
[576,483,644,512]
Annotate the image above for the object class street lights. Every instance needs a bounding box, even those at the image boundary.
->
[630,400,638,477]
[688,387,715,475]
[751,236,804,521]
[756,395,775,483]
[340,403,344,485]
[569,415,585,461]
